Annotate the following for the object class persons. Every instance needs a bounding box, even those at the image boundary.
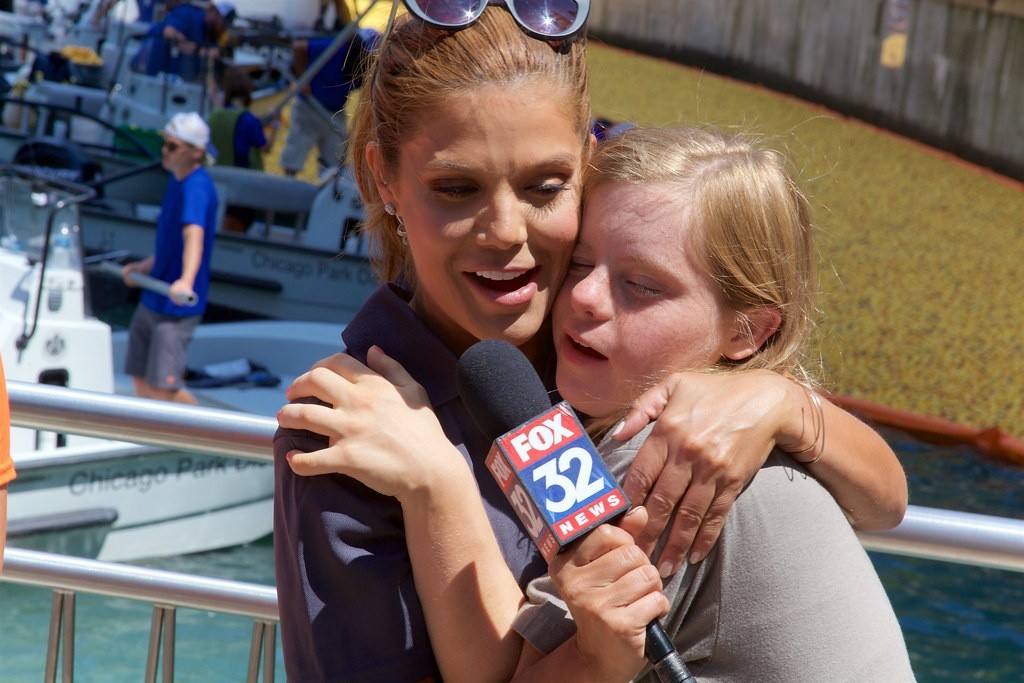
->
[277,129,916,683]
[122,112,218,406]
[90,0,406,183]
[275,0,908,683]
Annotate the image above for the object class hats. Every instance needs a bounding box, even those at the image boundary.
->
[165,112,219,160]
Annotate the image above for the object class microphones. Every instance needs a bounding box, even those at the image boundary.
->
[456,338,697,683]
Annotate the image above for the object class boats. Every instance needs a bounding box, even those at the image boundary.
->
[0,0,385,563]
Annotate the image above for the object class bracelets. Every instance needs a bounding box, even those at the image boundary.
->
[786,390,826,464]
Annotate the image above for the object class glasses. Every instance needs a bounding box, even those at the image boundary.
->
[370,0,591,103]
[160,142,178,151]
